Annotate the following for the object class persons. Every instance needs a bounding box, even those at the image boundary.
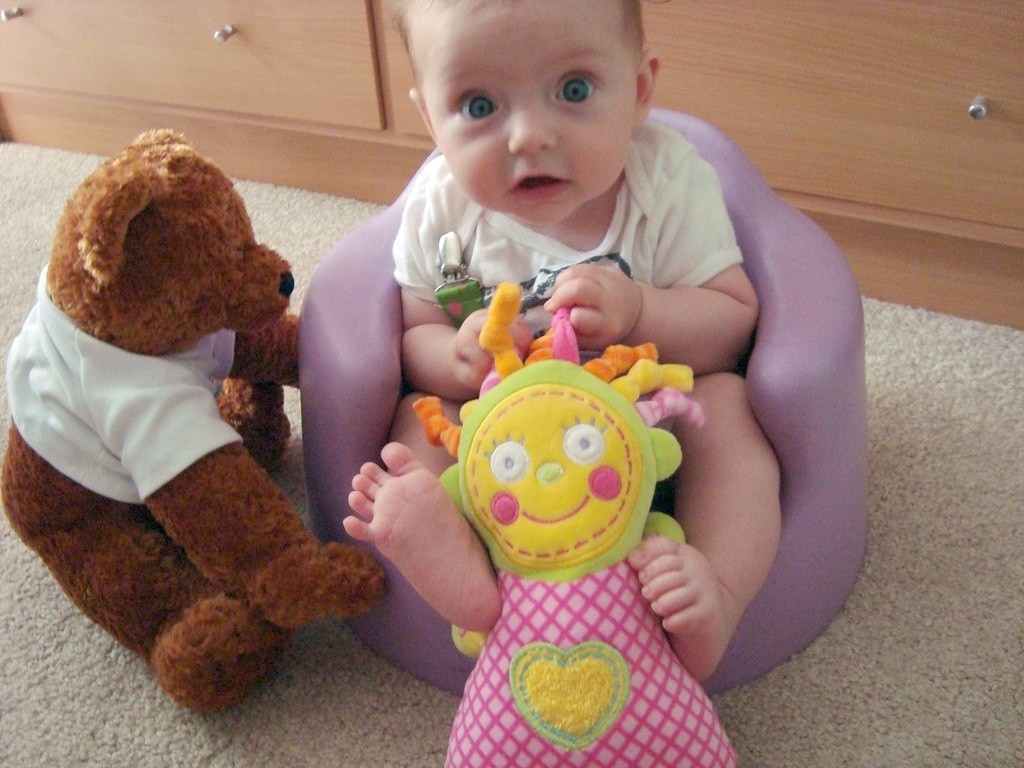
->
[341,0,780,684]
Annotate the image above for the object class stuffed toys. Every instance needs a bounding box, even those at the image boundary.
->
[414,283,743,768]
[0,129,387,708]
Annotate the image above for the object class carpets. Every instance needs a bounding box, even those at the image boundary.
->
[0,143,1024,768]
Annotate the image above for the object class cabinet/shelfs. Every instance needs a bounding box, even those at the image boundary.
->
[0,0,1024,331]
